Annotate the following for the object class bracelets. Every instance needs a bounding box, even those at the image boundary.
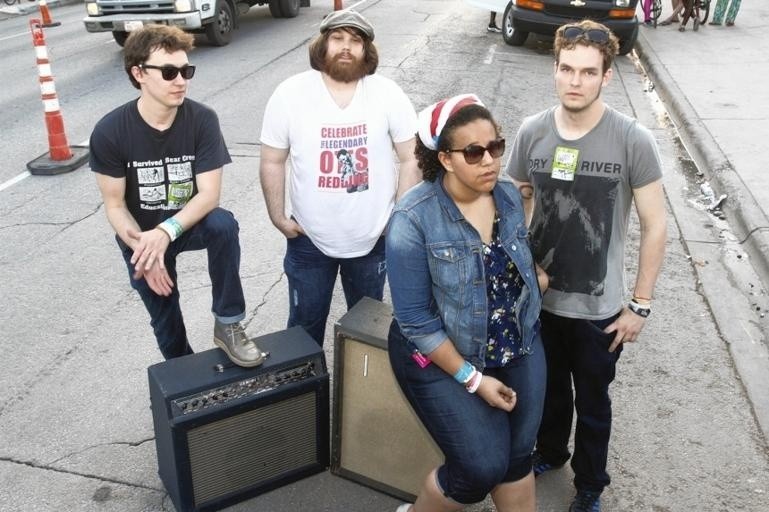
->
[628,299,651,318]
[632,294,652,301]
[154,217,184,243]
[454,360,483,394]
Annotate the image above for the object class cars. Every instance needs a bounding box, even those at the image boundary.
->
[500,0,638,55]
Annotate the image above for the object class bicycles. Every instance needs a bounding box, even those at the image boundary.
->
[641,0,663,31]
[684,0,711,24]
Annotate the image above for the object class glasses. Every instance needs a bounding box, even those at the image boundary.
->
[447,137,505,164]
[555,27,609,60]
[138,65,195,80]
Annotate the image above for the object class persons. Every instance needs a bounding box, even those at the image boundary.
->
[336,149,367,193]
[87,24,263,369]
[505,19,669,512]
[486,10,503,33]
[258,8,420,352]
[643,0,741,32]
[385,92,549,511]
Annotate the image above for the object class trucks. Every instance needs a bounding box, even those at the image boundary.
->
[83,0,311,46]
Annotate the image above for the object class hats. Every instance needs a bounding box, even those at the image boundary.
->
[320,10,375,40]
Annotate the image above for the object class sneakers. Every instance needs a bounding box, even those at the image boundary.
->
[569,492,601,512]
[532,450,564,477]
[214,319,262,367]
